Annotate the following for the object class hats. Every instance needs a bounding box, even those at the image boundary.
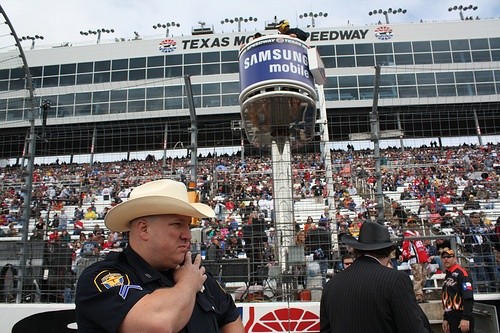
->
[341,219,401,251]
[441,249,454,256]
[104,179,216,232]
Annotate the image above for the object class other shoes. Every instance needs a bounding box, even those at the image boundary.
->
[417,300,428,303]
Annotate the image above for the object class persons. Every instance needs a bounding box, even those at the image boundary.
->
[438,248,476,333]
[0,141,500,302]
[319,219,435,333]
[73,178,247,333]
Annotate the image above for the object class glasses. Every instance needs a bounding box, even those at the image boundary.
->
[442,255,452,258]
[344,263,353,265]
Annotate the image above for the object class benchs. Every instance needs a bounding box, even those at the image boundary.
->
[16,184,500,226]
